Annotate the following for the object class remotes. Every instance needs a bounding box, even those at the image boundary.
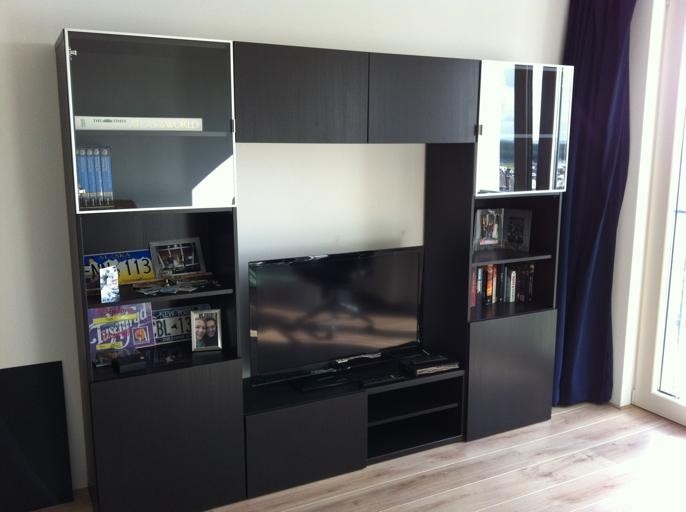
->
[360,374,405,387]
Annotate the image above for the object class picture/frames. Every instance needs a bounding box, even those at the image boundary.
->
[148,235,208,283]
[189,309,225,354]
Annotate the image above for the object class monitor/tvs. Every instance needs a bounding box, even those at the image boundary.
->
[248,245,424,392]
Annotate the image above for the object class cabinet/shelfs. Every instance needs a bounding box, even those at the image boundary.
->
[424,59,574,445]
[244,346,466,502]
[53,28,250,512]
[232,36,478,149]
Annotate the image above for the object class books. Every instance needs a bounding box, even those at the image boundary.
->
[470,260,538,309]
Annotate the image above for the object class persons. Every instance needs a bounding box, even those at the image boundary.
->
[194,317,207,347]
[74,143,117,211]
[201,317,218,346]
